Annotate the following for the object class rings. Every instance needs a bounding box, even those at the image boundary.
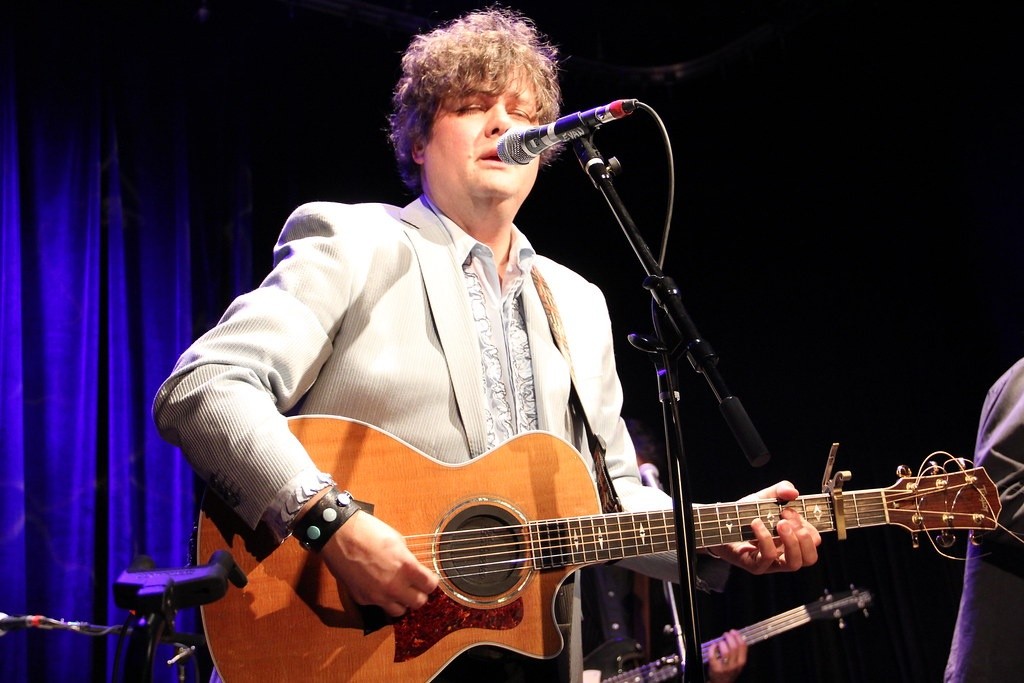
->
[720,657,728,664]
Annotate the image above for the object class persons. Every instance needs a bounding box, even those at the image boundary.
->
[580,565,747,683]
[152,11,823,683]
[945,358,1024,683]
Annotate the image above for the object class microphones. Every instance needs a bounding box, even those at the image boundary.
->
[0,611,48,637]
[496,98,637,165]
[640,463,658,490]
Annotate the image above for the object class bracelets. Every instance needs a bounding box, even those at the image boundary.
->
[292,485,360,554]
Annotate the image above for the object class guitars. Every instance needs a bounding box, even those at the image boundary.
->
[194,414,1003,683]
[582,583,871,683]
